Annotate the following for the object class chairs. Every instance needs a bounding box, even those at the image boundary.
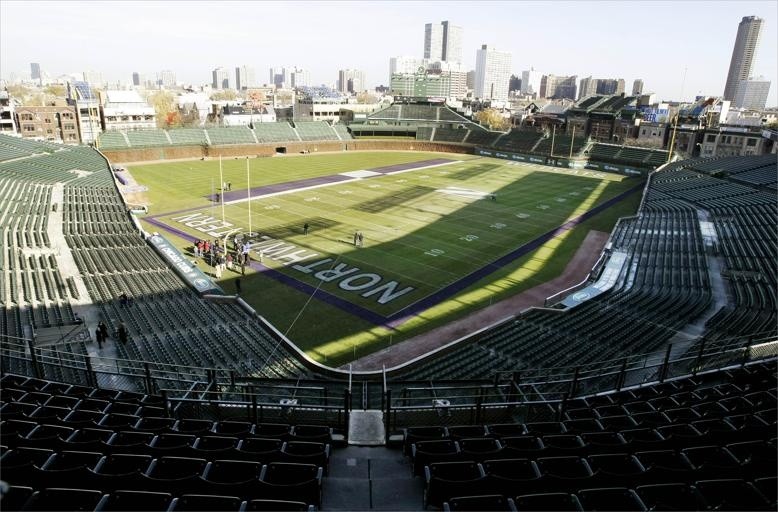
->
[388,297,778,512]
[0,133,147,248]
[97,120,331,149]
[330,95,670,163]
[0,373,331,512]
[1,246,316,379]
[546,151,778,305]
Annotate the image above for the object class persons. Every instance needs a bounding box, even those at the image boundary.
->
[193,233,258,294]
[353,232,358,247]
[95,293,132,350]
[212,180,232,201]
[358,232,364,249]
[304,223,310,236]
